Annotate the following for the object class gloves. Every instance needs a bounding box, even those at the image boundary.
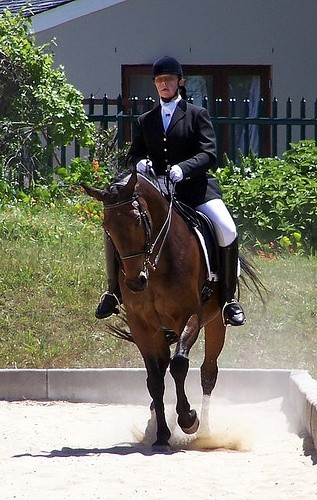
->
[166,165,183,182]
[137,159,152,173]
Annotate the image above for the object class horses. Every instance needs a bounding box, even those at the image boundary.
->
[79,163,271,455]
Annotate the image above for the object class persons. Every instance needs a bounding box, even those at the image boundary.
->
[94,56,245,328]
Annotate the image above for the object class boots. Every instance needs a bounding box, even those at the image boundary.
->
[95,232,122,318]
[217,234,245,326]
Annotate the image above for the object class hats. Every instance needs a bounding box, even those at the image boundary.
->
[152,56,183,80]
